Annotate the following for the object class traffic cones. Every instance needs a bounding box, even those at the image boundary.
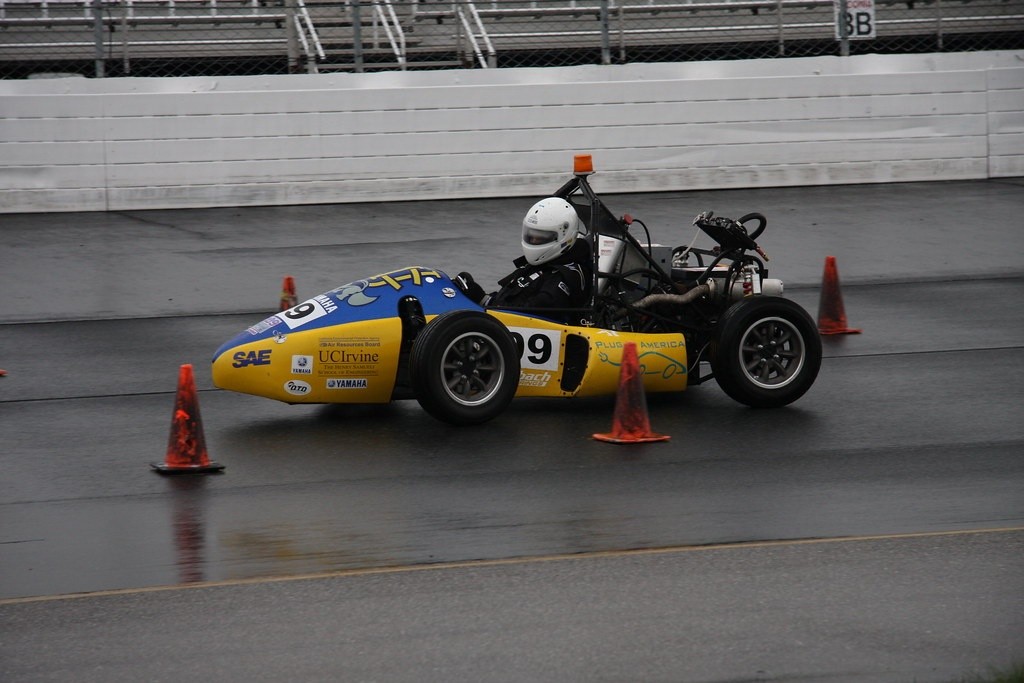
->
[148,363,228,475]
[594,341,675,445]
[818,255,866,335]
[277,274,298,315]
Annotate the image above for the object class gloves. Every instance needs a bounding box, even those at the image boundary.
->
[452,271,491,308]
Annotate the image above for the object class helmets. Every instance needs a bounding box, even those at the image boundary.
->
[521,197,579,265]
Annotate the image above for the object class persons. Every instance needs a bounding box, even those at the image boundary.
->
[453,197,588,321]
